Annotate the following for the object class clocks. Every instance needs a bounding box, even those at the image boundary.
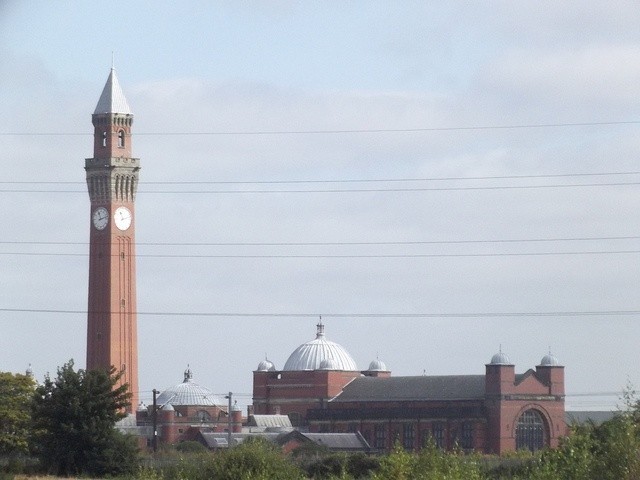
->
[114,206,133,231]
[92,206,110,232]
